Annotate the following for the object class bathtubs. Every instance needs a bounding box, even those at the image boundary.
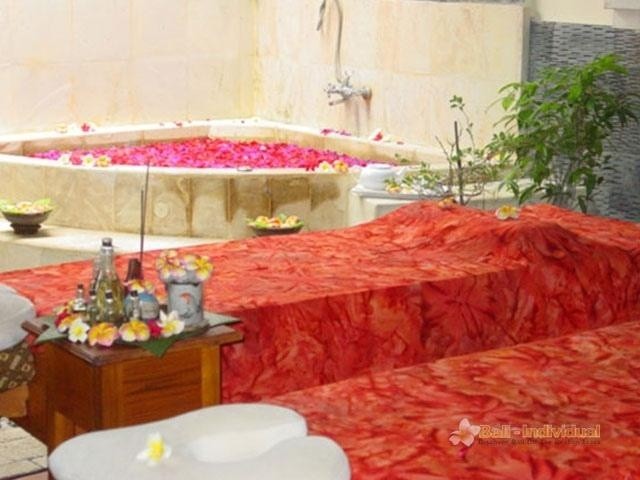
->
[0,118,520,242]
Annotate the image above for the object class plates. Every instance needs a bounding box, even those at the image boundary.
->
[249,222,303,237]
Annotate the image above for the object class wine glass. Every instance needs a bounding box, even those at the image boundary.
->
[0,205,54,235]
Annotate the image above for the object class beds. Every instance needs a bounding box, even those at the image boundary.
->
[0,199,640,480]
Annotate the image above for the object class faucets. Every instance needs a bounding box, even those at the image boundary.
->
[313,0,373,108]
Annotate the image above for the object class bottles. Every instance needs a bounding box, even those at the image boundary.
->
[100,288,120,327]
[72,282,87,313]
[87,290,102,329]
[91,236,125,321]
[126,290,142,322]
